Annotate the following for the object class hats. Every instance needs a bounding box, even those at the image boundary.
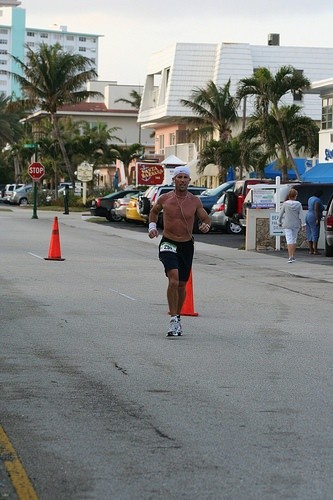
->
[173,166,189,178]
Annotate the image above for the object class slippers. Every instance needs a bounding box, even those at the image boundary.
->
[309,251,314,254]
[314,251,321,255]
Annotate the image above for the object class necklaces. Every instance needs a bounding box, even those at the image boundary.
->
[173,192,187,198]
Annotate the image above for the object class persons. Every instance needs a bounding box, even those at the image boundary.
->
[305,189,324,255]
[278,189,303,263]
[148,166,211,336]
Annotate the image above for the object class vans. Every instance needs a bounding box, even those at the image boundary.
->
[243,181,333,222]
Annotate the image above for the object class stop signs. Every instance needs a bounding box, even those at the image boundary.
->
[27,162,45,181]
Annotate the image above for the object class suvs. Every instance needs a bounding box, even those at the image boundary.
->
[223,177,296,237]
[0,182,73,206]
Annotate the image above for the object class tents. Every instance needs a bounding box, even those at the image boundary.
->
[300,163,333,184]
[249,157,318,180]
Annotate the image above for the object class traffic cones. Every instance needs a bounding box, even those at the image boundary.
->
[167,264,200,317]
[43,216,66,262]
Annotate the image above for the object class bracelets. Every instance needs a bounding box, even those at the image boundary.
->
[149,222,156,229]
[207,224,210,228]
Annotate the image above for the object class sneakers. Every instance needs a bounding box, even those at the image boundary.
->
[178,322,184,335]
[167,319,179,336]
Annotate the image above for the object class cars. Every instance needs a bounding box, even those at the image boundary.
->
[208,194,243,234]
[321,196,333,256]
[113,183,209,227]
[191,179,238,231]
[89,190,149,223]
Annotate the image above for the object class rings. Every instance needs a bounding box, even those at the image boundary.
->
[207,230,208,231]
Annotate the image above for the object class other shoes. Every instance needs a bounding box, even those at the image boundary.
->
[292,258,296,261]
[287,258,292,263]
